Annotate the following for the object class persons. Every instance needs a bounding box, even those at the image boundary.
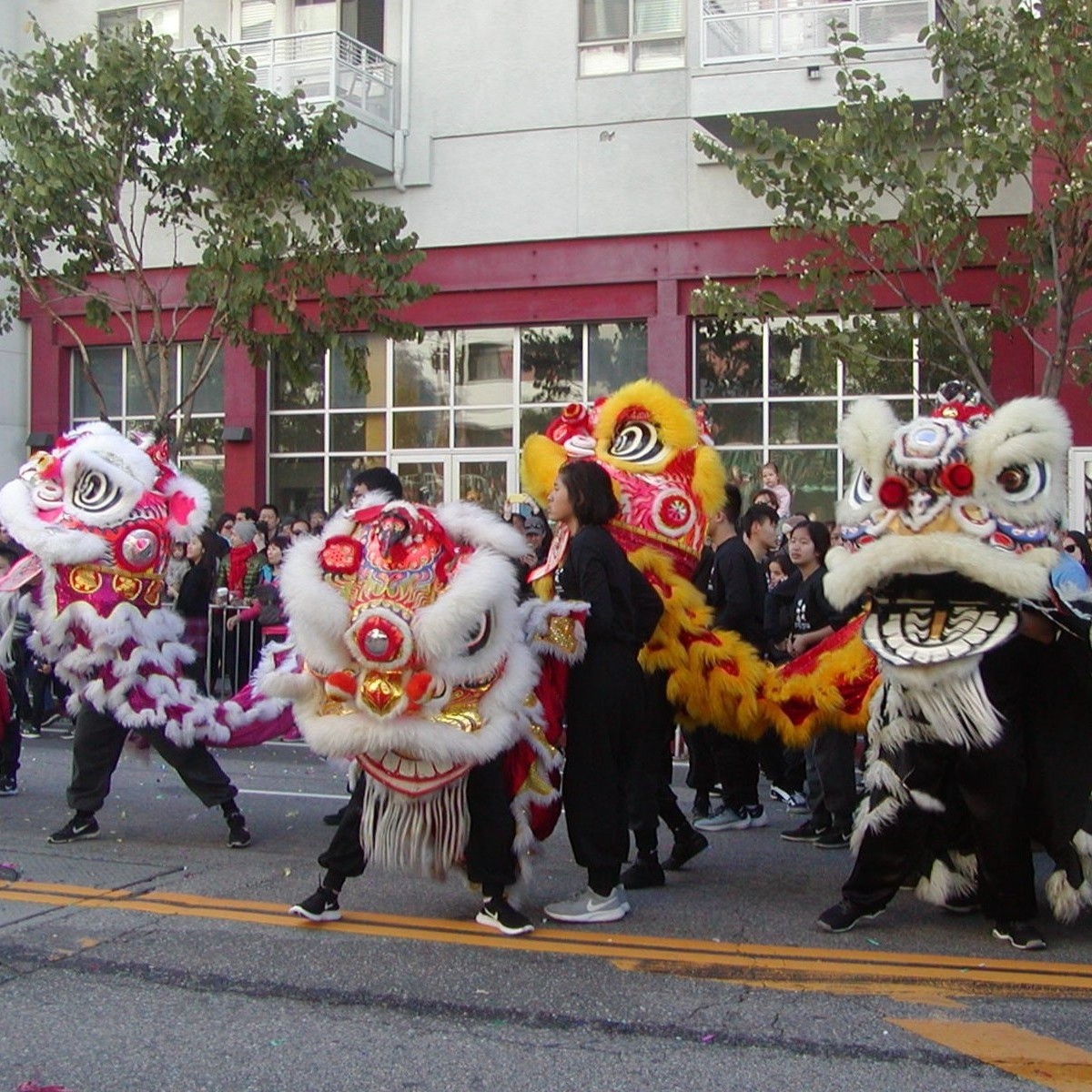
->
[46,691,252,848]
[174,527,217,698]
[620,532,710,891]
[290,466,536,935]
[813,604,1047,949]
[694,483,768,832]
[781,521,859,848]
[503,492,552,607]
[545,461,665,923]
[1052,528,1092,575]
[0,525,79,796]
[743,463,875,810]
[161,504,329,695]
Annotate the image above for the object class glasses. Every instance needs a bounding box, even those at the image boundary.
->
[291,529,302,536]
[524,522,543,529]
[222,524,233,529]
[1063,544,1078,553]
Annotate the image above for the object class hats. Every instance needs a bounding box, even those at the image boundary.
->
[524,517,545,536]
[232,520,256,544]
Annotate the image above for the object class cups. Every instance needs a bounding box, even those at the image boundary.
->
[217,588,228,604]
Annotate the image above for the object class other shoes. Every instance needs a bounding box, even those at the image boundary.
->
[660,832,710,871]
[691,796,712,825]
[16,702,78,741]
[0,776,19,795]
[621,850,666,888]
[281,725,303,742]
[944,895,979,912]
[325,809,342,825]
[900,877,920,891]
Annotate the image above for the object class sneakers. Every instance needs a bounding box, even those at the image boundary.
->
[813,828,853,847]
[817,898,885,931]
[746,803,768,828]
[47,815,100,842]
[769,782,808,814]
[544,878,630,922]
[287,872,341,921]
[778,818,831,841]
[992,912,1048,949]
[475,894,536,935]
[694,804,752,831]
[225,813,250,847]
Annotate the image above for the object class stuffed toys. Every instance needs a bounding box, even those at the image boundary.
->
[931,379,993,429]
[521,378,884,750]
[823,395,1092,920]
[0,420,302,749]
[282,489,591,911]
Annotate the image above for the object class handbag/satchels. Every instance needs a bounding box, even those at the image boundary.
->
[254,583,290,626]
